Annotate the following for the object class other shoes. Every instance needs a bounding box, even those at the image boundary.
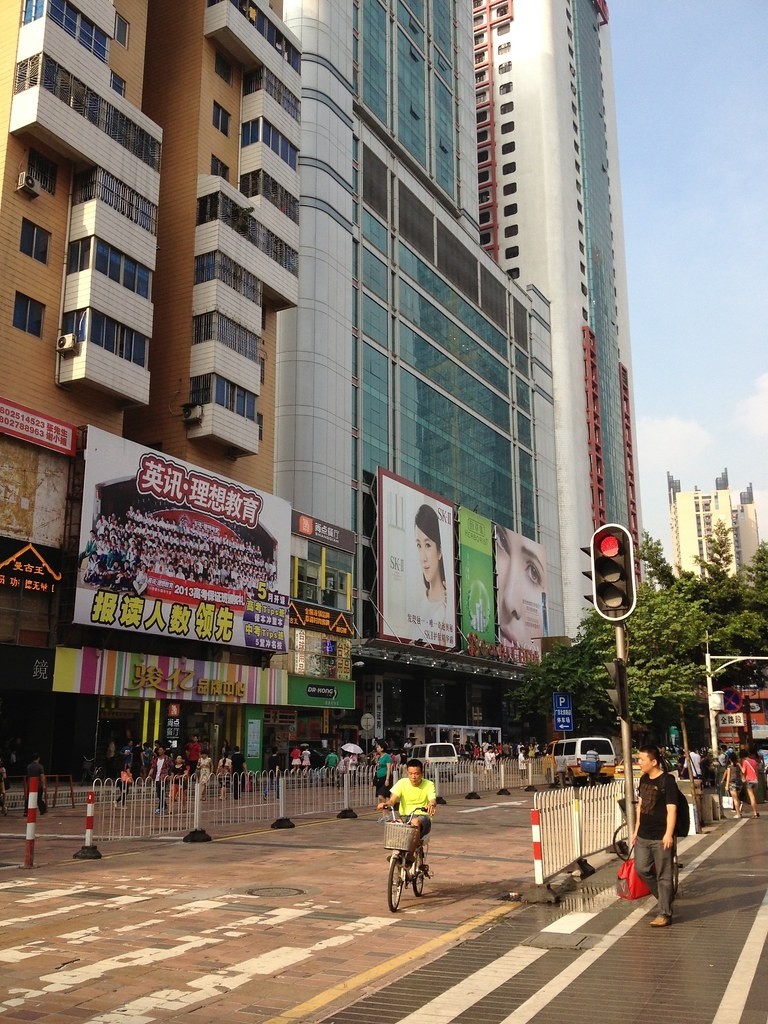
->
[155,809,160,814]
[40,810,48,815]
[386,854,402,864]
[752,815,757,818]
[648,915,672,926]
[405,852,416,868]
[23,812,28,816]
[113,800,117,808]
[121,806,128,809]
[733,815,741,818]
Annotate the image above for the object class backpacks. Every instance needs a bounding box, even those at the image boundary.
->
[638,773,691,837]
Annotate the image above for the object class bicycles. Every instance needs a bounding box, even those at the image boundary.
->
[588,772,596,789]
[383,805,434,913]
[613,797,683,899]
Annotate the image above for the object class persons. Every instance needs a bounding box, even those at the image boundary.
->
[105,731,365,814]
[392,735,600,790]
[377,758,437,869]
[23,753,48,817]
[414,504,455,648]
[0,756,8,816]
[376,741,393,824]
[726,752,743,819]
[736,749,760,820]
[495,524,546,668]
[78,505,280,607]
[632,743,679,927]
[660,744,768,789]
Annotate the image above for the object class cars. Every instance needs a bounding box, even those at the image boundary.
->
[614,754,672,783]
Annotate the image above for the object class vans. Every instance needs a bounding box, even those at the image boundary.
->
[542,738,618,788]
[405,743,459,782]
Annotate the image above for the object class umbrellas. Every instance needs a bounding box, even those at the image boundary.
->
[341,743,363,754]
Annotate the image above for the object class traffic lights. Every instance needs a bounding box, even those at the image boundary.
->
[589,524,638,622]
[603,660,625,711]
[580,546,594,605]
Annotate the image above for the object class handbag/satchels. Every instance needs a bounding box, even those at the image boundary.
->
[120,770,133,785]
[246,773,251,792]
[697,775,706,785]
[722,790,733,810]
[616,845,651,900]
[373,775,377,786]
